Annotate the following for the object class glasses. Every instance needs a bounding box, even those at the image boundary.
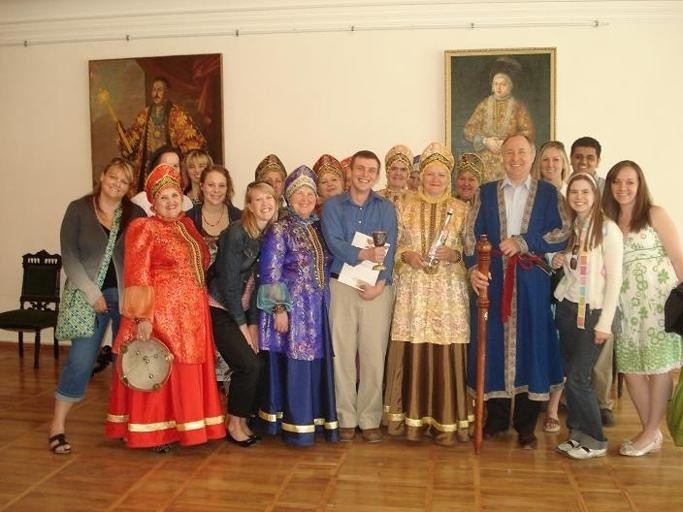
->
[570,245,579,269]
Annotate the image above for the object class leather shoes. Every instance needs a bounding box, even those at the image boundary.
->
[518,432,537,450]
[618,429,663,457]
[248,434,262,442]
[471,431,504,440]
[226,427,257,447]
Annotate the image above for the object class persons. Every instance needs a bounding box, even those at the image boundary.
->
[119,75,217,200]
[602,160,682,457]
[139,133,617,448]
[547,173,626,457]
[462,56,536,183]
[50,158,148,455]
[108,166,227,456]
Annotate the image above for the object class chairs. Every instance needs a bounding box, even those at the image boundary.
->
[0,249,62,368]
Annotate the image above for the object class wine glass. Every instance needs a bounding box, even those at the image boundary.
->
[371,230,388,270]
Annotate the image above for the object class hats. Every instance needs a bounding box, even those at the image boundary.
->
[490,56,523,82]
[253,142,487,200]
[145,163,185,204]
[412,155,420,173]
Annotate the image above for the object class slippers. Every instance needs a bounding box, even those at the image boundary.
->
[543,417,560,432]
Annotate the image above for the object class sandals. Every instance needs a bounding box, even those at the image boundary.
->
[49,433,71,454]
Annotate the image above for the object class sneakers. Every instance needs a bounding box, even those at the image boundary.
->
[600,409,615,426]
[566,444,606,459]
[338,428,355,442]
[362,429,382,440]
[555,439,580,455]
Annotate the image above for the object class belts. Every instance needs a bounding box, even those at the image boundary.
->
[330,272,339,279]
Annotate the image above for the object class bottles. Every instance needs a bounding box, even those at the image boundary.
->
[426,207,453,278]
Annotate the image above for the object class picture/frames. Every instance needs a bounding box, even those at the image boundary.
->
[444,47,557,185]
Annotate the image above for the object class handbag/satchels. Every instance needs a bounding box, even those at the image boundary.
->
[54,288,96,341]
[666,365,683,447]
